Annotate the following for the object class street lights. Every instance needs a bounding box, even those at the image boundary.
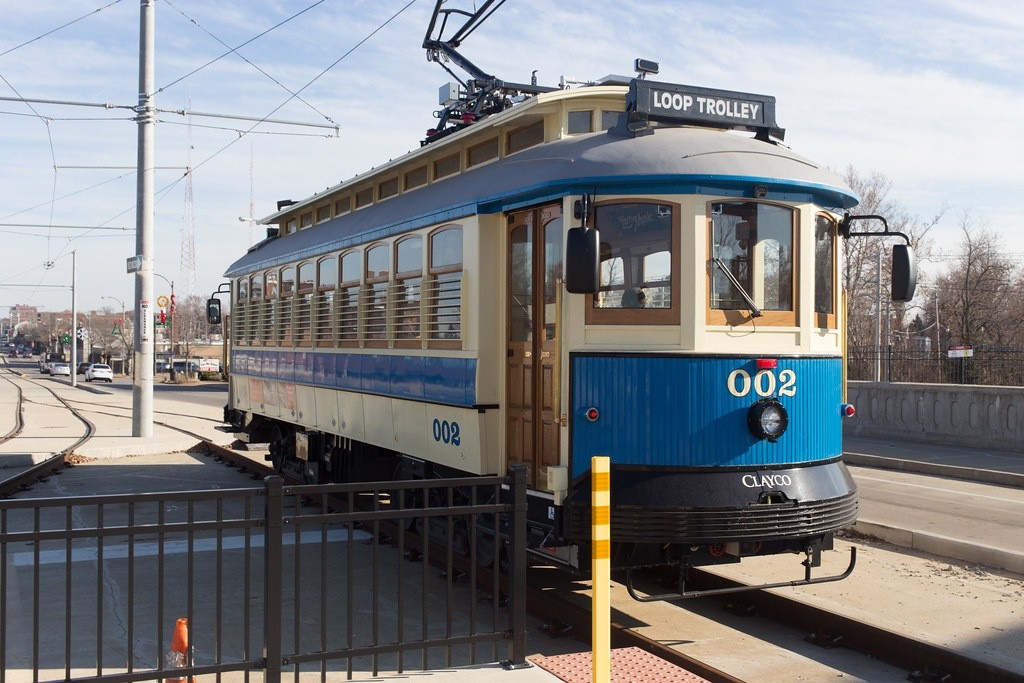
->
[101,296,125,376]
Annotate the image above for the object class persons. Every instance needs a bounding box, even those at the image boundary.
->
[620,286,646,308]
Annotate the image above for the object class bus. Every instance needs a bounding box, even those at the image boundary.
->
[199,1,920,607]
[38,351,66,374]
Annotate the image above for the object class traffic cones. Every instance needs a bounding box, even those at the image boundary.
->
[161,618,197,683]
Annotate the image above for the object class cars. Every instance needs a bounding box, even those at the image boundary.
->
[155,360,200,373]
[22,350,33,358]
[84,363,114,383]
[48,361,71,377]
[9,349,18,358]
[77,362,92,375]
